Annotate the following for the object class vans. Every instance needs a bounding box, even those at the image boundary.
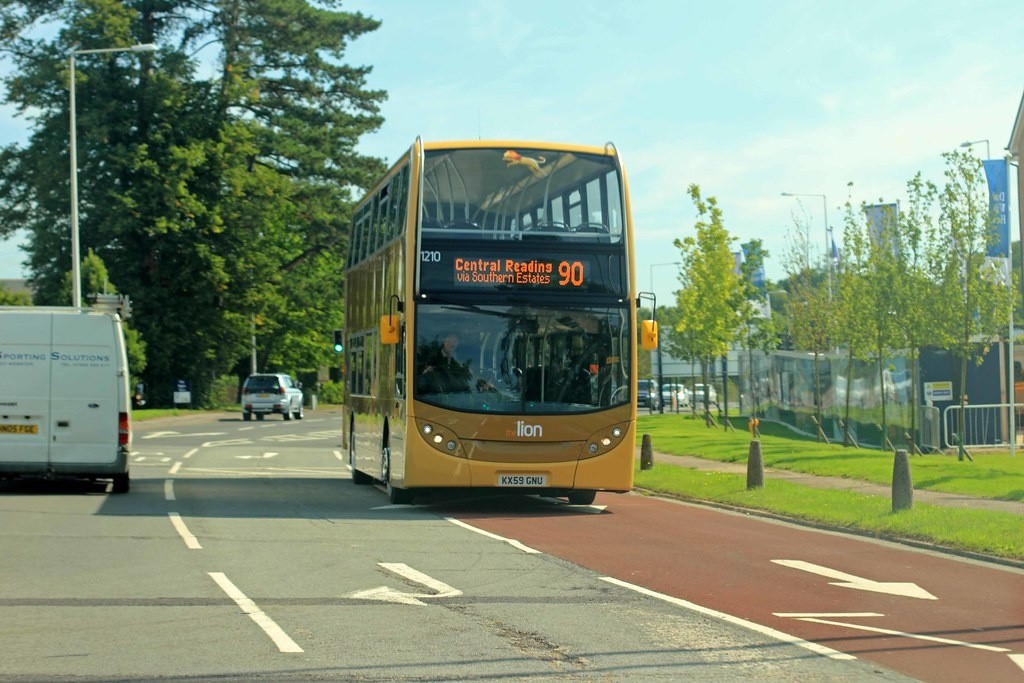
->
[1,304,134,495]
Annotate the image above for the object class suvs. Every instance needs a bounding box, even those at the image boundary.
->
[637,380,659,411]
[241,373,304,420]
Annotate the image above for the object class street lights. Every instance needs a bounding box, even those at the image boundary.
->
[650,261,681,319]
[781,192,834,304]
[68,44,161,306]
[960,138,991,159]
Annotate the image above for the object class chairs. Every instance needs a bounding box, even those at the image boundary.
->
[422,221,611,243]
[480,368,592,404]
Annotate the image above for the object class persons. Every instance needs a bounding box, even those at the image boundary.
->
[883,367,892,385]
[419,332,460,374]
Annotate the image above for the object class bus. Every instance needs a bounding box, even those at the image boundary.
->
[340,135,658,506]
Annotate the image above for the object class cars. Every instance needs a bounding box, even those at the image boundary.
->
[689,383,717,404]
[658,383,690,407]
